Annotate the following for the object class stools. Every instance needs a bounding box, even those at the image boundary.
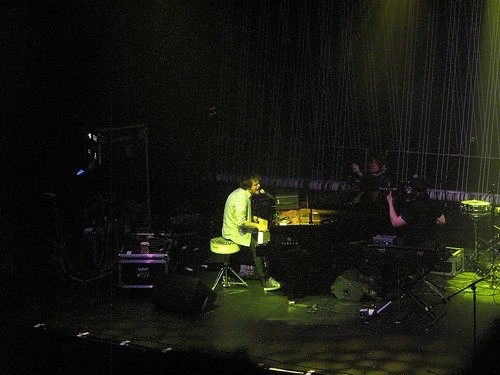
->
[208,237,252,291]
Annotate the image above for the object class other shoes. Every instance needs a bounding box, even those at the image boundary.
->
[363,284,376,297]
[360,273,372,284]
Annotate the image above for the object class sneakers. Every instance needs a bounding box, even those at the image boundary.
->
[264,281,280,291]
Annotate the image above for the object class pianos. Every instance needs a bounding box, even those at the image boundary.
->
[366,234,447,256]
[257,202,339,300]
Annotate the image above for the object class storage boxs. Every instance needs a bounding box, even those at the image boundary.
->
[118,238,178,290]
[275,189,299,210]
[430,247,466,277]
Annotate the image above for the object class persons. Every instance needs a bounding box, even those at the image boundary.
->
[352,159,390,192]
[366,177,446,304]
[220,169,284,293]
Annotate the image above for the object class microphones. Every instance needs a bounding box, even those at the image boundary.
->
[260,189,276,201]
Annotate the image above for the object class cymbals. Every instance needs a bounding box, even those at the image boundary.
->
[461,199,491,206]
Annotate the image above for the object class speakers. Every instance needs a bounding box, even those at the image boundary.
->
[161,275,218,317]
